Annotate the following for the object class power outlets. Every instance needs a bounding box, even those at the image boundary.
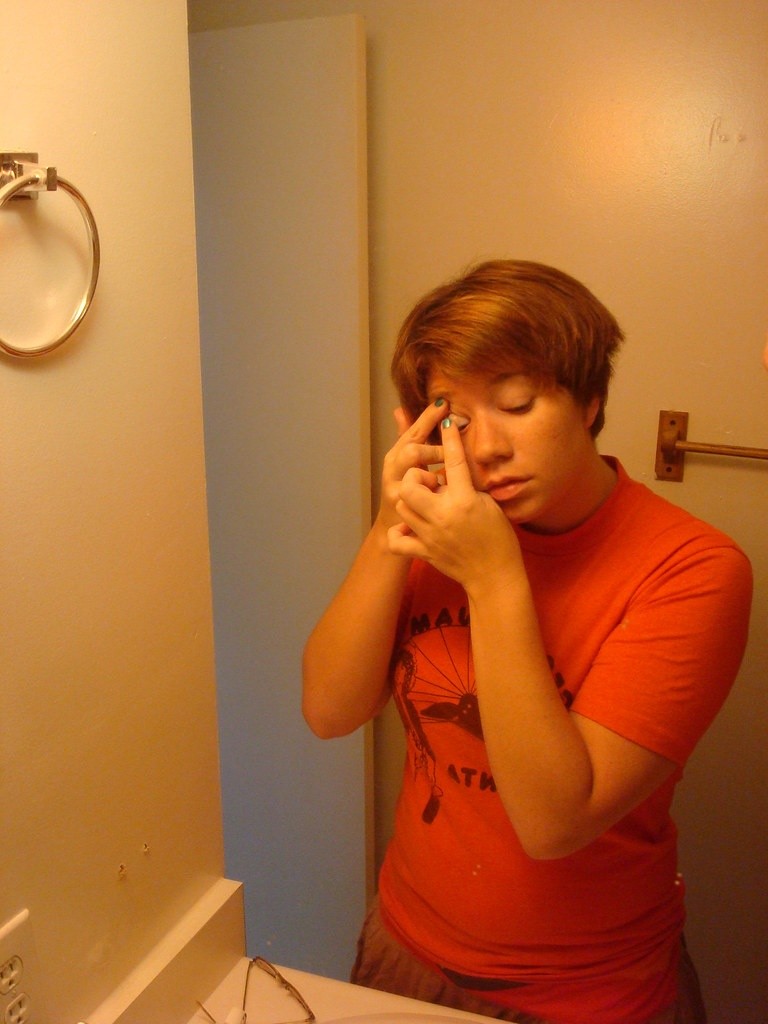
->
[0,909,44,1024]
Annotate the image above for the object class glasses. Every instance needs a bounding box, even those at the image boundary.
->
[196,956,315,1024]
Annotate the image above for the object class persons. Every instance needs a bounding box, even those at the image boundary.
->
[301,260,753,1024]
